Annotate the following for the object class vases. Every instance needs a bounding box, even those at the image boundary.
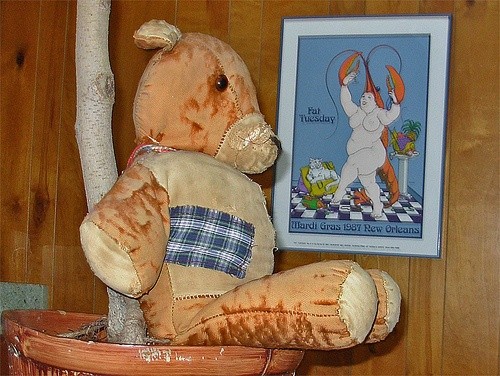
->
[1,309,307,376]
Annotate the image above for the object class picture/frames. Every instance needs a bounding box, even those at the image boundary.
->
[270,12,453,258]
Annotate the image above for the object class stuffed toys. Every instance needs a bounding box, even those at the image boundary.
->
[78,18,403,351]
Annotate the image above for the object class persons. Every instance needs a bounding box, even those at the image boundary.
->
[330,71,401,218]
